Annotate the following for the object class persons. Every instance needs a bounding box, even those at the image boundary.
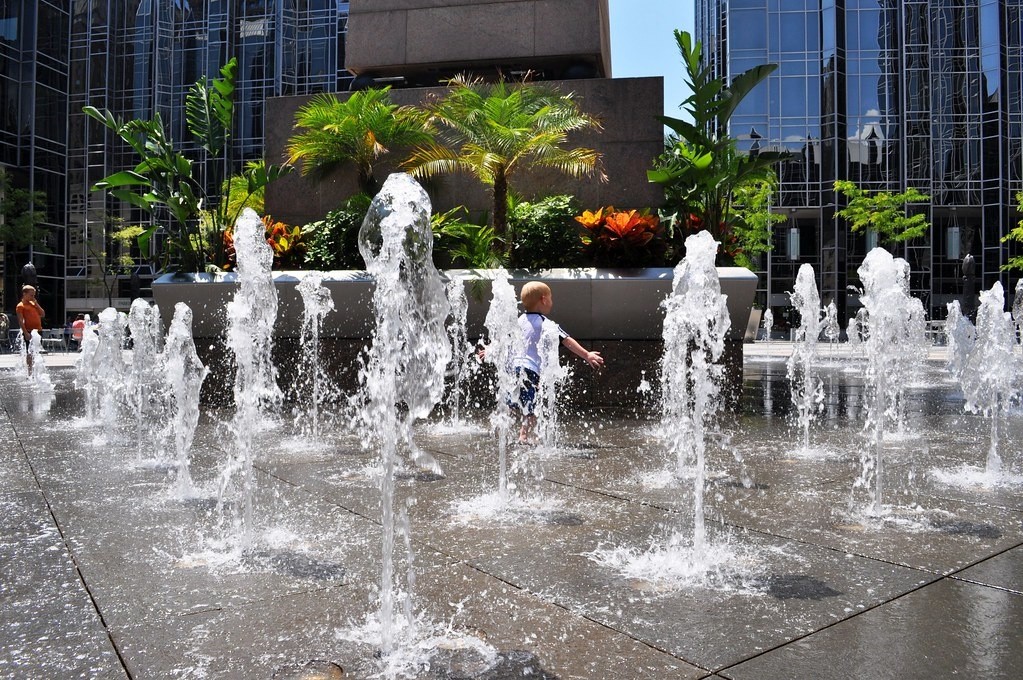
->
[16,286,45,379]
[0,313,8,354]
[64,313,99,352]
[479,281,603,446]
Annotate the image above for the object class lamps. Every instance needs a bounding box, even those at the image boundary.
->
[866,222,878,253]
[947,207,960,260]
[785,208,801,260]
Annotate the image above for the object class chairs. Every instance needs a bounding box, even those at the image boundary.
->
[8,327,84,354]
